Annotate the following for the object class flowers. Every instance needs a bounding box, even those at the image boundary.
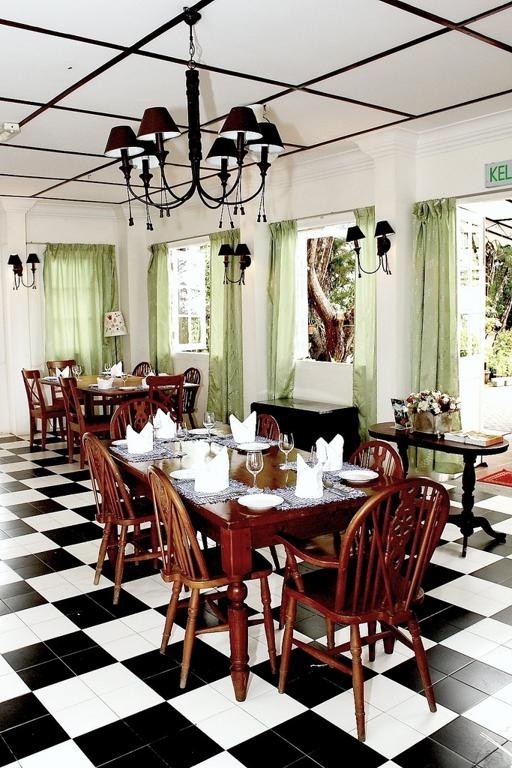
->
[406,391,461,416]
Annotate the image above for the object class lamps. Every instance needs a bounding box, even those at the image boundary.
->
[103,310,128,365]
[7,254,40,291]
[218,243,251,286]
[344,220,394,278]
[104,10,285,229]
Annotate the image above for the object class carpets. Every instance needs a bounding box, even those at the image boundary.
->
[475,468,511,487]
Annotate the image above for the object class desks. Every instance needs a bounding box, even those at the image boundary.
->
[367,421,508,558]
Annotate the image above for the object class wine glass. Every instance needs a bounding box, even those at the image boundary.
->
[149,415,160,445]
[104,363,110,378]
[121,373,128,388]
[278,433,294,472]
[72,364,82,382]
[204,412,216,441]
[245,450,264,493]
[175,420,187,456]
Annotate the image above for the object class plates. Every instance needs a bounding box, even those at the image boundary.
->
[169,469,195,483]
[188,428,212,435]
[236,442,269,451]
[338,469,379,484]
[89,383,99,386]
[237,493,284,511]
[119,386,137,390]
[111,439,127,446]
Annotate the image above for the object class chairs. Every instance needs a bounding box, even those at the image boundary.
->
[21,359,201,469]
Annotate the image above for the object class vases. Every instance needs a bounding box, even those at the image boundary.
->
[409,409,462,439]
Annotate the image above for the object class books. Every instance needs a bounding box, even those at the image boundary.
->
[444,430,471,444]
[465,433,503,447]
